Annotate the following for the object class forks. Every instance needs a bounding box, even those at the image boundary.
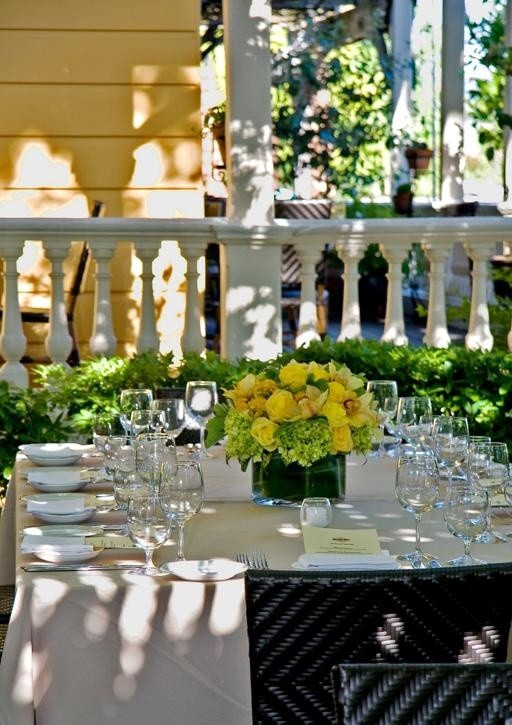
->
[237,552,269,570]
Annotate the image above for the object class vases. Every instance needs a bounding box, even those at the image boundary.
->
[250,449,347,505]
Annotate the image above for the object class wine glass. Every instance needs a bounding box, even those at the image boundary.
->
[366,380,512,567]
[91,381,217,577]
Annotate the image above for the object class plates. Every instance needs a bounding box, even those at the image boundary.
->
[159,558,248,582]
[33,482,88,492]
[27,455,82,466]
[18,493,94,504]
[14,466,81,475]
[32,547,100,565]
[36,508,98,523]
[18,526,103,539]
[15,442,81,450]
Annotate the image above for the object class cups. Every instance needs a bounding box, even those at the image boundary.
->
[300,496,332,529]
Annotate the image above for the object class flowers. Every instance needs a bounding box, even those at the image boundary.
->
[204,357,386,471]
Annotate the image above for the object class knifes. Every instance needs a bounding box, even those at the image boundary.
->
[19,562,146,575]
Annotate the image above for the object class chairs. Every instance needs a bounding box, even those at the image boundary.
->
[277,200,335,346]
[242,562,511,724]
[328,661,511,725]
[1,198,108,367]
